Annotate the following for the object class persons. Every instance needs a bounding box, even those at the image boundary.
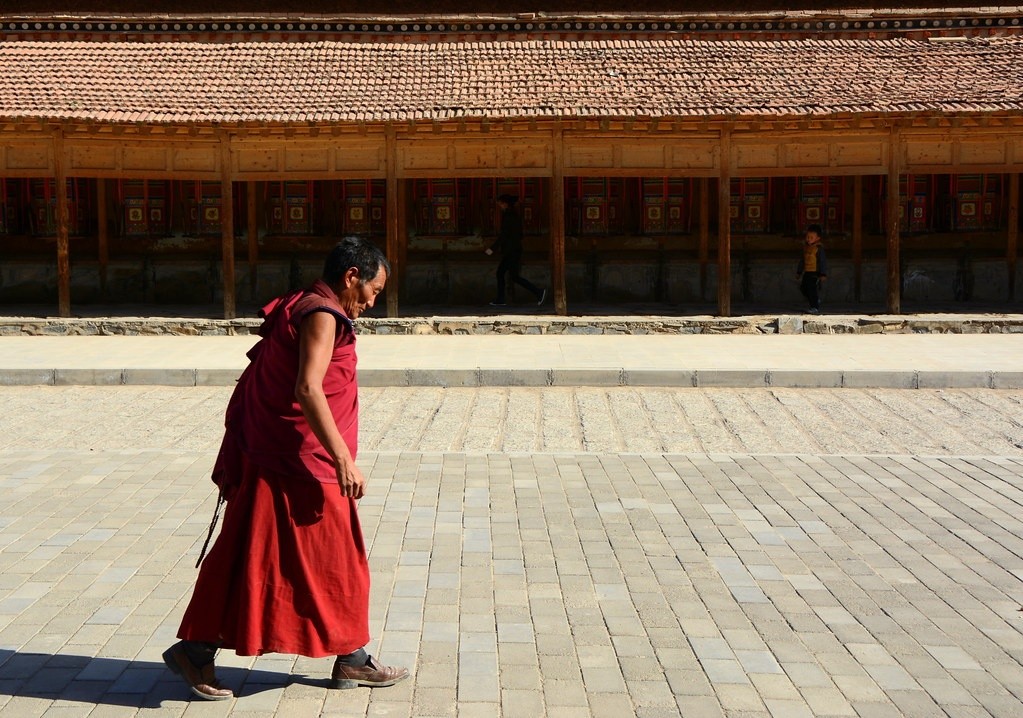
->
[162,237,409,700]
[485,194,546,306]
[796,225,827,314]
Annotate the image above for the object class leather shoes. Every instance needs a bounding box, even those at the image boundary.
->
[162,643,234,700]
[332,655,410,689]
